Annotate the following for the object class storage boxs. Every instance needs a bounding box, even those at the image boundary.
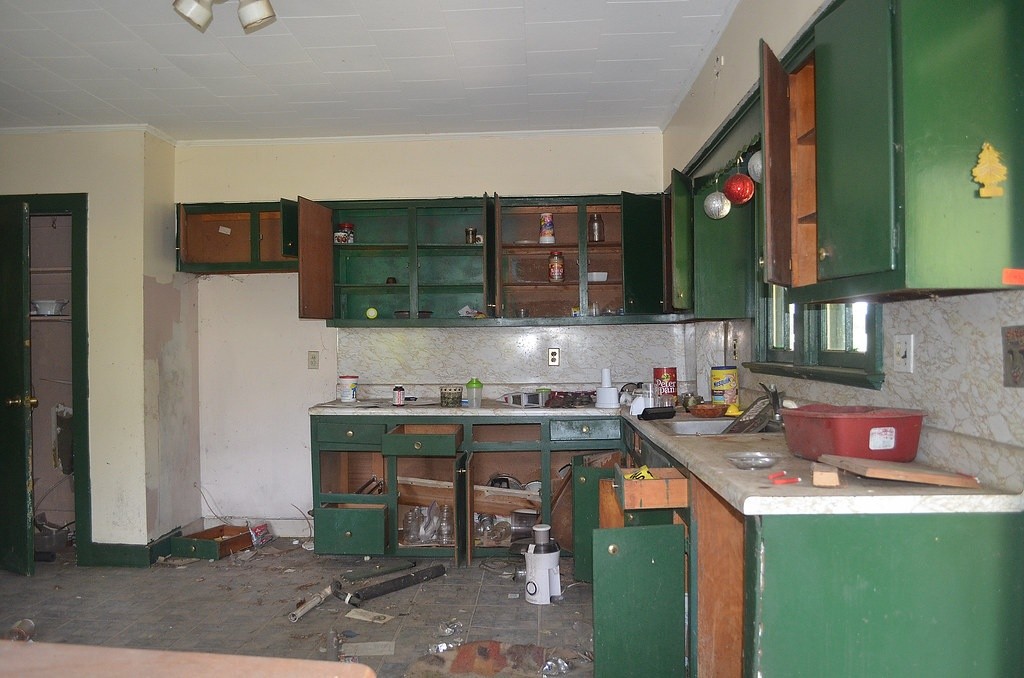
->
[777,403,928,463]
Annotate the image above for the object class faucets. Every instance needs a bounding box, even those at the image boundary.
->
[758,382,780,414]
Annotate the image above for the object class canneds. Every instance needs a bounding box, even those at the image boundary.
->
[548,251,564,282]
[652,367,677,405]
[5,619,35,641]
[710,365,739,408]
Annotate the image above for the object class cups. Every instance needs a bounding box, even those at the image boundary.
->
[538,212,556,244]
[464,227,477,244]
[640,382,676,409]
[516,309,528,317]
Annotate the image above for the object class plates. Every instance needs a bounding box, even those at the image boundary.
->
[394,310,434,318]
[587,272,609,282]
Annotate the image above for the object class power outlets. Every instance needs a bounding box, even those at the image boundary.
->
[308,351,319,368]
[1001,326,1024,387]
[893,334,913,373]
[548,348,560,366]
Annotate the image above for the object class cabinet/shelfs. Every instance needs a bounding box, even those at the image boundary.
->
[175,0,1024,328]
[30,267,72,321]
[308,414,1024,678]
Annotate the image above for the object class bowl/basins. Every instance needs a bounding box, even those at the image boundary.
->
[725,450,783,470]
[31,300,69,315]
[688,402,729,417]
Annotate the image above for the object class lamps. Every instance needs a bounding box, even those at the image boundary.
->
[173,0,276,32]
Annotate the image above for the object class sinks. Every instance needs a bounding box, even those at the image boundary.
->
[649,416,786,437]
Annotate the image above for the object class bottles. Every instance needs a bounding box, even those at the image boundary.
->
[548,253,565,283]
[588,214,605,242]
[334,222,355,245]
[466,377,483,408]
[404,505,512,544]
